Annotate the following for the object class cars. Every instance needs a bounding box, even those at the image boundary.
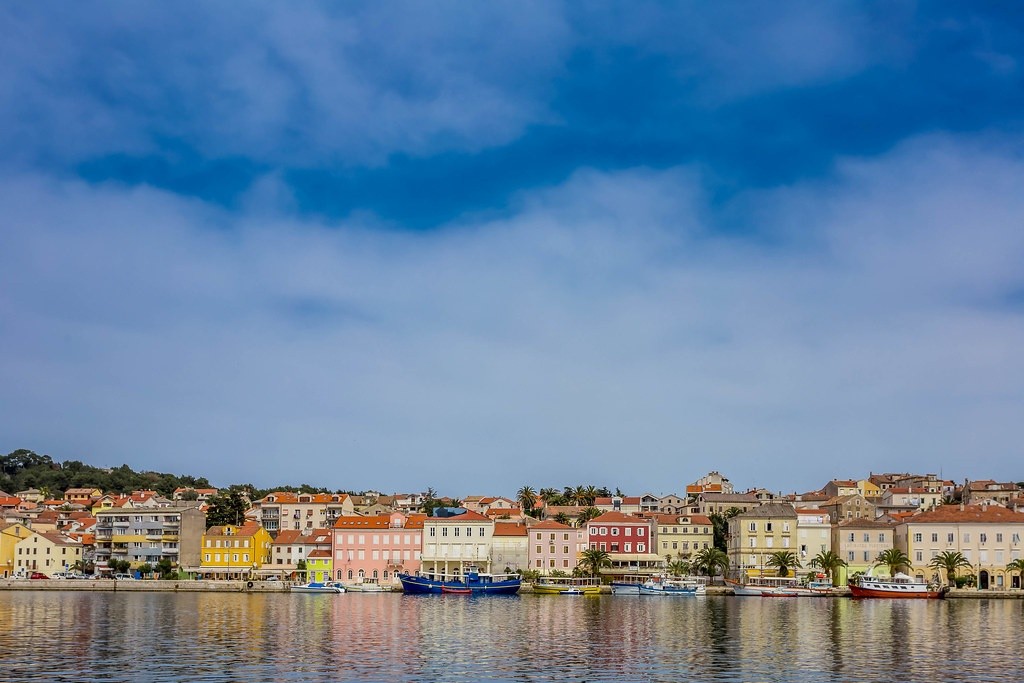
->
[30,572,50,579]
[50,571,137,580]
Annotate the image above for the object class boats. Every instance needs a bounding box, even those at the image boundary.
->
[720,579,853,596]
[848,560,951,600]
[396,565,524,594]
[610,571,699,595]
[289,580,345,594]
[441,584,472,594]
[346,576,394,593]
[531,576,602,594]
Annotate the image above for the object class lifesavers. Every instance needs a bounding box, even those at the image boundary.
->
[247,581,254,588]
